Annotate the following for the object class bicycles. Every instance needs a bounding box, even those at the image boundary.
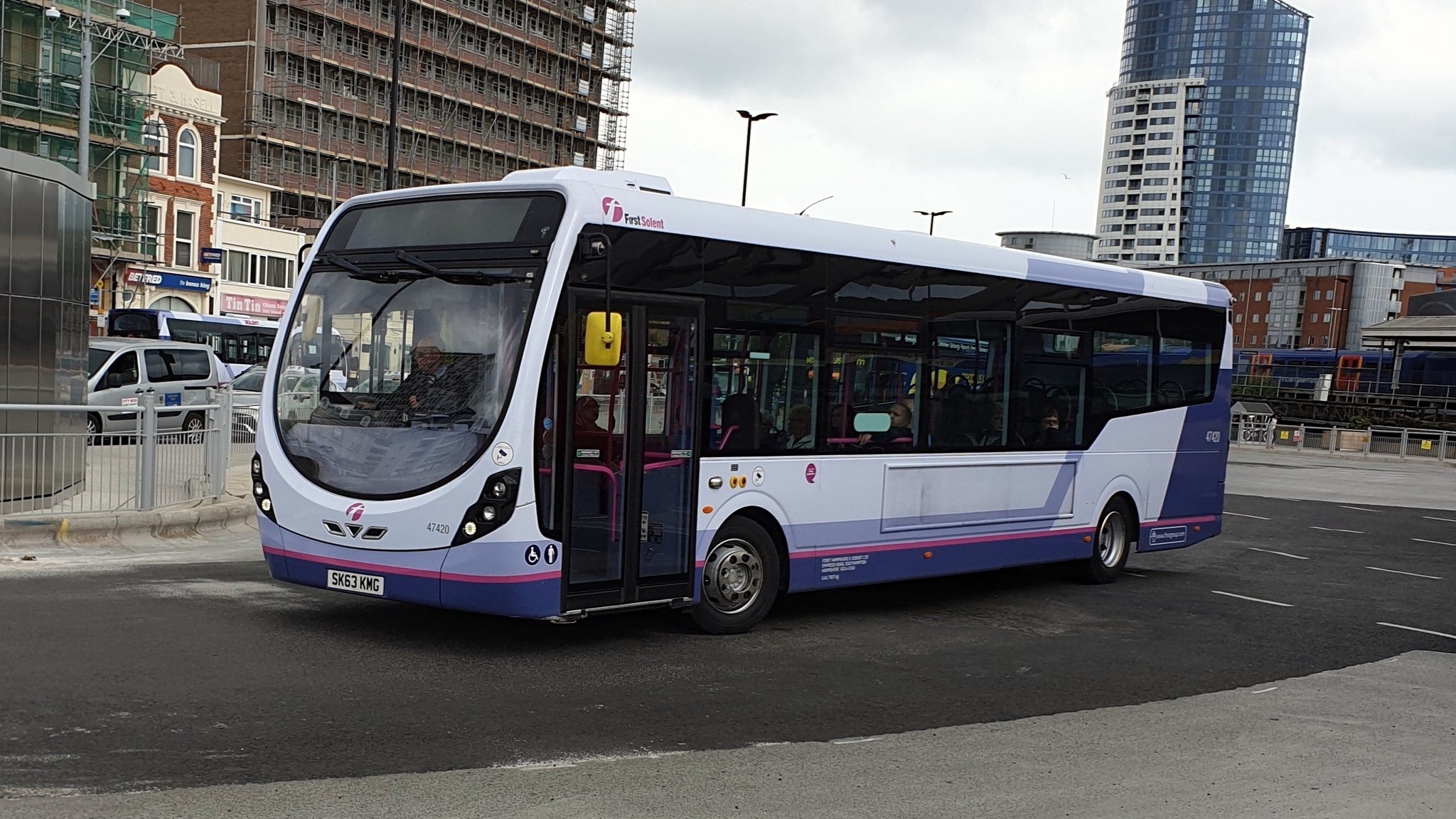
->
[1240,415,1274,442]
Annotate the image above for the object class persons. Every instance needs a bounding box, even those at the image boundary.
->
[1266,413,1281,450]
[573,377,1060,473]
[353,337,468,410]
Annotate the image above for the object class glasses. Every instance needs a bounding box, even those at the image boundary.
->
[412,350,429,358]
[786,418,802,424]
[583,405,601,412]
[1040,419,1059,425]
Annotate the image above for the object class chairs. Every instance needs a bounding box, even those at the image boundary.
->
[762,376,1185,448]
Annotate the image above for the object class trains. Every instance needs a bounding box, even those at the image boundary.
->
[1091,342,1455,424]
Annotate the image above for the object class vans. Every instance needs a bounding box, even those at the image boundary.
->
[87,337,221,445]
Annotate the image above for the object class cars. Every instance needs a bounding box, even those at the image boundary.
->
[650,382,666,396]
[215,362,402,443]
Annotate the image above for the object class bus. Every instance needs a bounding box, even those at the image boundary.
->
[106,309,348,389]
[250,165,1235,635]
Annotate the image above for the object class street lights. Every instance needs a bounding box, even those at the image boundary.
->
[912,208,953,236]
[1326,307,1347,347]
[736,110,778,207]
[1324,277,1348,430]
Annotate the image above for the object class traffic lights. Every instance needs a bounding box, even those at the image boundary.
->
[658,359,665,374]
